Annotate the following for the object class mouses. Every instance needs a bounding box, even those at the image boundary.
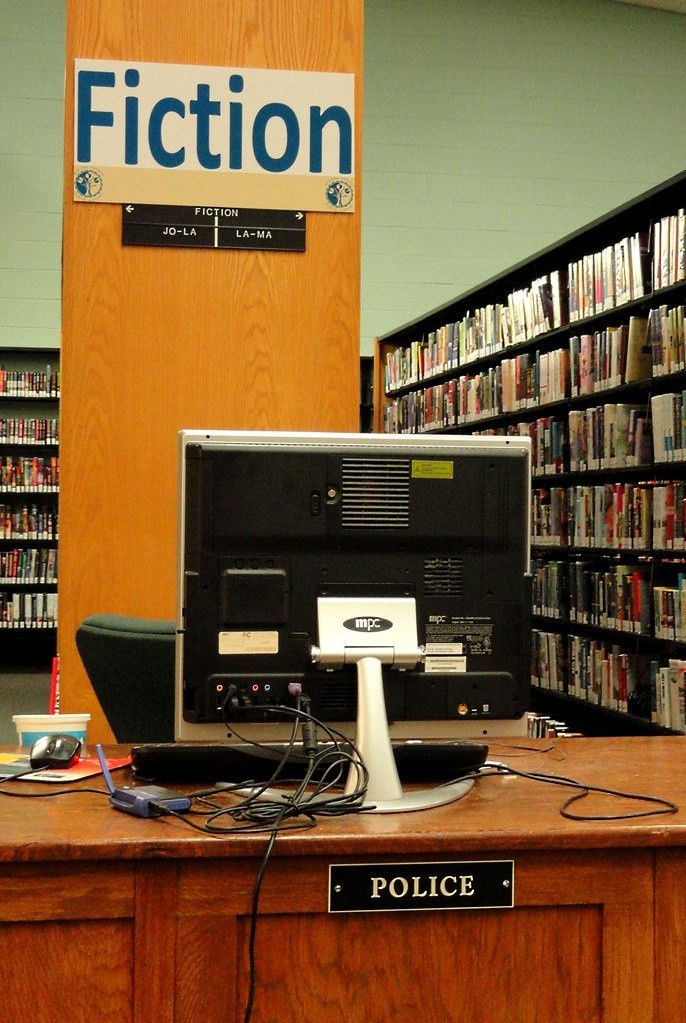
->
[30,734,81,769]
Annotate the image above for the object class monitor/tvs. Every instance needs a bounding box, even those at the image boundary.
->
[176,430,532,741]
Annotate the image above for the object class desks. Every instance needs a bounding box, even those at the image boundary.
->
[0,739,686,1023]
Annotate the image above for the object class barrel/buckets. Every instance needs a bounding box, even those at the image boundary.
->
[12,713,91,748]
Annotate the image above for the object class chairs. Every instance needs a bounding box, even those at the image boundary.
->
[77,613,188,739]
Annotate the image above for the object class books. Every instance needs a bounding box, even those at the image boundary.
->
[383,204,686,739]
[1,361,62,633]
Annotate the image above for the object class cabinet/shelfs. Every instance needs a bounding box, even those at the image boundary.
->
[0,342,60,671]
[367,166,685,726]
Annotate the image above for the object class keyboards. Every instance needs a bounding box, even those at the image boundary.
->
[130,743,486,782]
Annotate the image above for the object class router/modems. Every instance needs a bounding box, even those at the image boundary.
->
[97,744,192,818]
